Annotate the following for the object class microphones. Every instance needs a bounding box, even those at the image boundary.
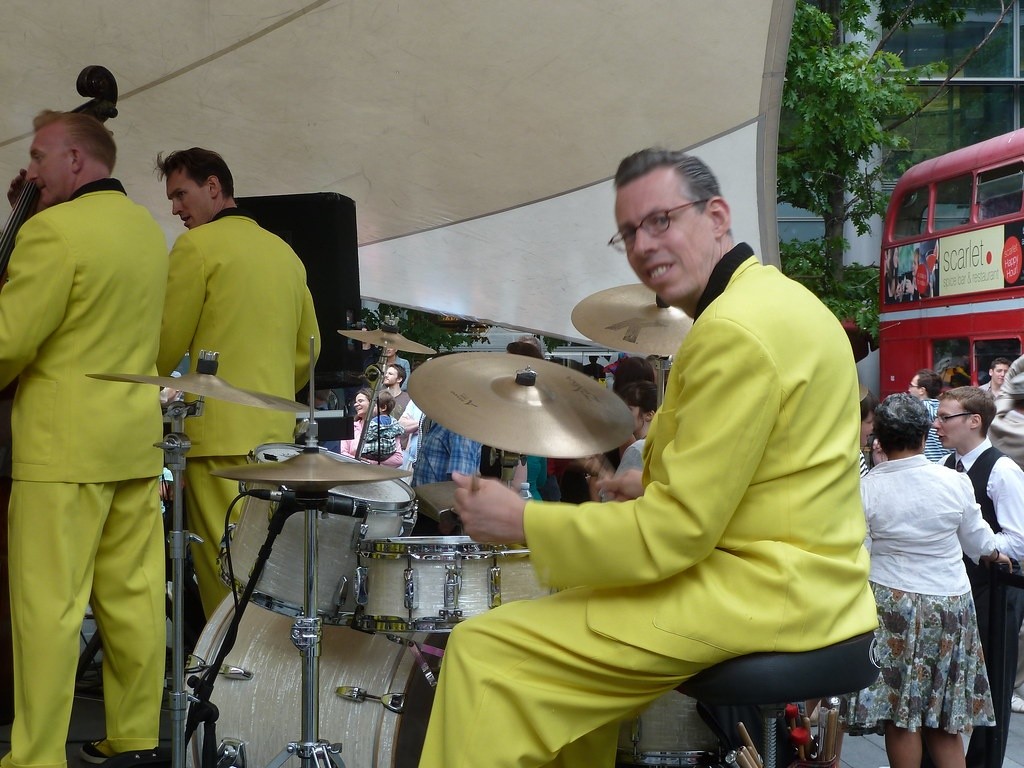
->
[248,489,367,518]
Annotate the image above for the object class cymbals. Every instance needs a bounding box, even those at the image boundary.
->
[209,452,412,497]
[84,369,314,414]
[335,325,438,356]
[570,281,695,357]
[406,351,634,459]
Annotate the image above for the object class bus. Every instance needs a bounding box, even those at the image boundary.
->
[875,126,1024,406]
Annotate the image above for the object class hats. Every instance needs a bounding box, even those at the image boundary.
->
[1000,355,1024,395]
[507,336,542,358]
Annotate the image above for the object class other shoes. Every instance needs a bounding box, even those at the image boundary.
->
[1010,695,1024,713]
[80,737,109,764]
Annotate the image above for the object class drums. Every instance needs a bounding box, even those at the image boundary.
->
[352,534,569,638]
[173,590,450,768]
[618,682,730,768]
[216,442,418,625]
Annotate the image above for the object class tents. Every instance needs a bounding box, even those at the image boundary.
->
[0,1,796,358]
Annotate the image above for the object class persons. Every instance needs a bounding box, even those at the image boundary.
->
[979,357,1012,401]
[419,146,878,768]
[313,328,658,505]
[883,247,930,304]
[909,369,956,463]
[988,353,1024,472]
[156,147,320,622]
[859,383,879,479]
[845,392,1013,768]
[933,386,1024,768]
[0,109,170,768]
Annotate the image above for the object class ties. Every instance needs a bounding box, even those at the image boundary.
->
[956,460,963,473]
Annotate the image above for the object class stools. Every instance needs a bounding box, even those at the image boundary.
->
[671,631,880,768]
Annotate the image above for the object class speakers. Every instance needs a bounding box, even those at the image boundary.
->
[239,192,366,393]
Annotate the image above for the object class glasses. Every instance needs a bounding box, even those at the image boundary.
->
[909,384,919,388]
[933,412,971,424]
[608,199,707,252]
